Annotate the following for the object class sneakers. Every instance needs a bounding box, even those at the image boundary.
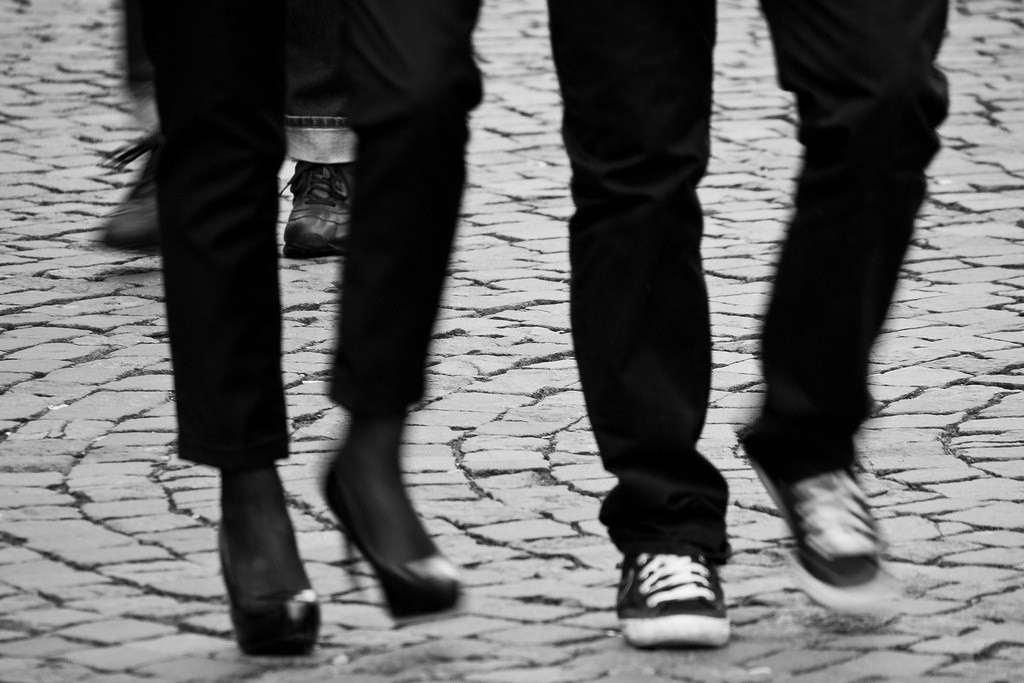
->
[615,551,733,648]
[101,133,164,244]
[279,159,353,253]
[746,452,881,613]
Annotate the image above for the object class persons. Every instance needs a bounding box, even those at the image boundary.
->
[101,0,952,655]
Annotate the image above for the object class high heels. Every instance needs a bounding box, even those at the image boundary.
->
[325,453,459,618]
[217,522,321,654]
